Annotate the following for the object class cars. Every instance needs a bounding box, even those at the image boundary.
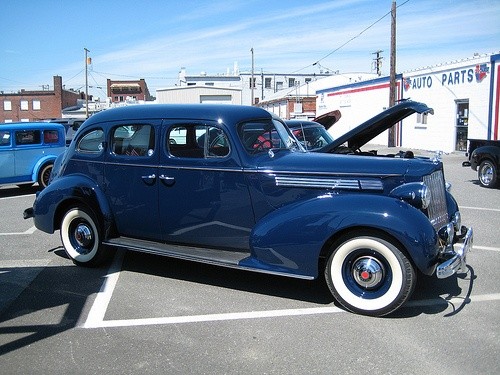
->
[280,99,434,162]
[25,102,473,318]
[0,122,66,189]
[464,137,500,189]
[47,117,131,166]
